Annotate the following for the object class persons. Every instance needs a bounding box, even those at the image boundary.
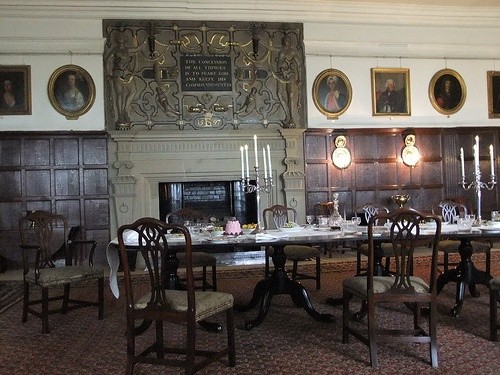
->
[256,36,302,129]
[106,30,146,129]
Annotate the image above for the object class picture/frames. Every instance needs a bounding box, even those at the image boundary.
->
[428,68,467,116]
[370,67,412,117]
[312,68,352,120]
[0,64,32,116]
[48,64,96,120]
[486,71,500,119]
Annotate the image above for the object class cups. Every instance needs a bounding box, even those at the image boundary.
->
[491,211,500,222]
[457,214,475,233]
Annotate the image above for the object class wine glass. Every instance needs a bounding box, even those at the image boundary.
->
[318,214,362,235]
[306,215,314,224]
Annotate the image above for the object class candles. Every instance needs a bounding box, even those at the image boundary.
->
[244,144,250,177]
[472,144,479,175]
[475,135,480,171]
[253,134,259,167]
[262,147,268,178]
[459,147,465,176]
[266,144,273,177]
[239,145,245,178]
[489,144,494,175]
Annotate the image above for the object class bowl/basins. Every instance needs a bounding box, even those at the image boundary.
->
[241,228,255,233]
[281,226,304,233]
[211,230,225,237]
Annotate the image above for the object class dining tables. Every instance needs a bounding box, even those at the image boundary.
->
[106,222,500,338]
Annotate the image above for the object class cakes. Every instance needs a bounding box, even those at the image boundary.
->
[223,220,243,235]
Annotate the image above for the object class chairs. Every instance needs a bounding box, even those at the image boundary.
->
[341,206,442,369]
[166,209,218,292]
[354,202,413,277]
[19,210,105,335]
[263,205,322,292]
[431,200,493,275]
[117,217,236,375]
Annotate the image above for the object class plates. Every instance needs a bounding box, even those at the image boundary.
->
[426,221,449,228]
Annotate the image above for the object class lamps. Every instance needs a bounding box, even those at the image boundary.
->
[331,136,352,170]
[401,134,422,168]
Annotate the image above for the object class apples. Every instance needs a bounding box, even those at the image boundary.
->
[242,224,256,229]
